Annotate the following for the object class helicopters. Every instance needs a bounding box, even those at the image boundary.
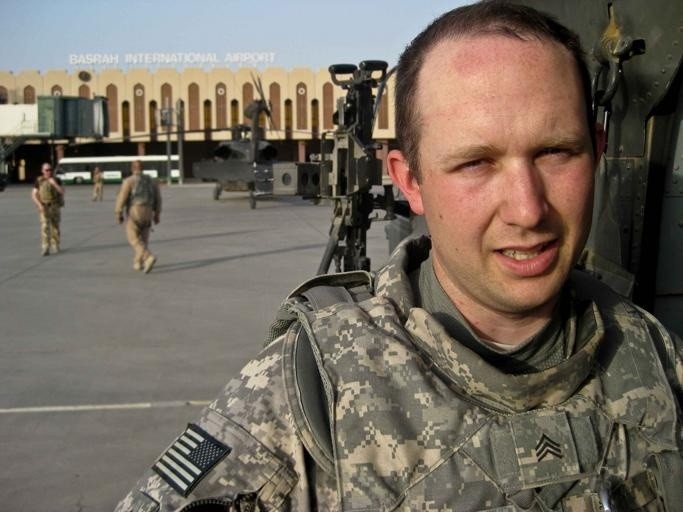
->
[69,71,332,209]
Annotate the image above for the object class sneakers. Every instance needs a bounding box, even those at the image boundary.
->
[131,262,143,271]
[53,246,60,254]
[40,246,50,256]
[143,254,156,274]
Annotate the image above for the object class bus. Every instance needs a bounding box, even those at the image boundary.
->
[47,152,182,186]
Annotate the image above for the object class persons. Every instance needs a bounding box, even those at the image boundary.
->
[111,1,682,512]
[113,160,161,275]
[90,166,103,203]
[30,163,65,257]
